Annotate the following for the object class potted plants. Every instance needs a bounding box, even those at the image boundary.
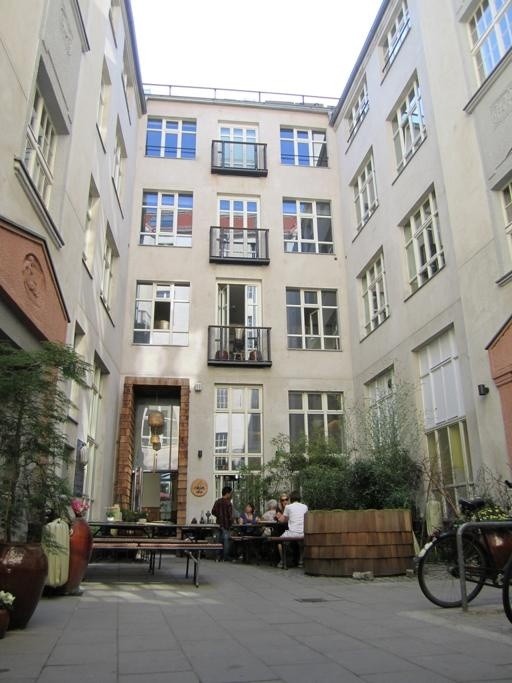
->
[102,506,147,560]
[0,340,95,629]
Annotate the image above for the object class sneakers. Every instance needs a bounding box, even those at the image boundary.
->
[277,562,284,568]
[298,562,304,568]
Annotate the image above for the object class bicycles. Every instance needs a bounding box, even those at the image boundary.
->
[411,480,512,608]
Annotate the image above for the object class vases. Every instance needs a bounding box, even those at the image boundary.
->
[480,530,512,578]
[65,519,92,595]
[0,609,10,639]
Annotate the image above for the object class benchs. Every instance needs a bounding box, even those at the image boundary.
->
[92,536,304,587]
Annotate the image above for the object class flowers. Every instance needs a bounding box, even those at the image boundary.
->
[449,505,512,530]
[0,590,19,611]
[65,494,94,516]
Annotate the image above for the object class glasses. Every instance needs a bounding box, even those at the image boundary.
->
[281,498,289,502]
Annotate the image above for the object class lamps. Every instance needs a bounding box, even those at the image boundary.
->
[148,397,164,479]
[478,385,489,395]
[198,451,202,457]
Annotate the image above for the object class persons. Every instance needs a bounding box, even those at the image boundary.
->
[231,504,239,525]
[271,492,290,568]
[258,499,279,537]
[276,490,309,568]
[211,486,235,562]
[239,503,260,535]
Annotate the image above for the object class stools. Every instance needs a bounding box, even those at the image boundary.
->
[250,350,262,360]
[216,351,228,360]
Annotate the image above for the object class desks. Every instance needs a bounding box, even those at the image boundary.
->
[231,523,288,536]
[89,522,220,541]
